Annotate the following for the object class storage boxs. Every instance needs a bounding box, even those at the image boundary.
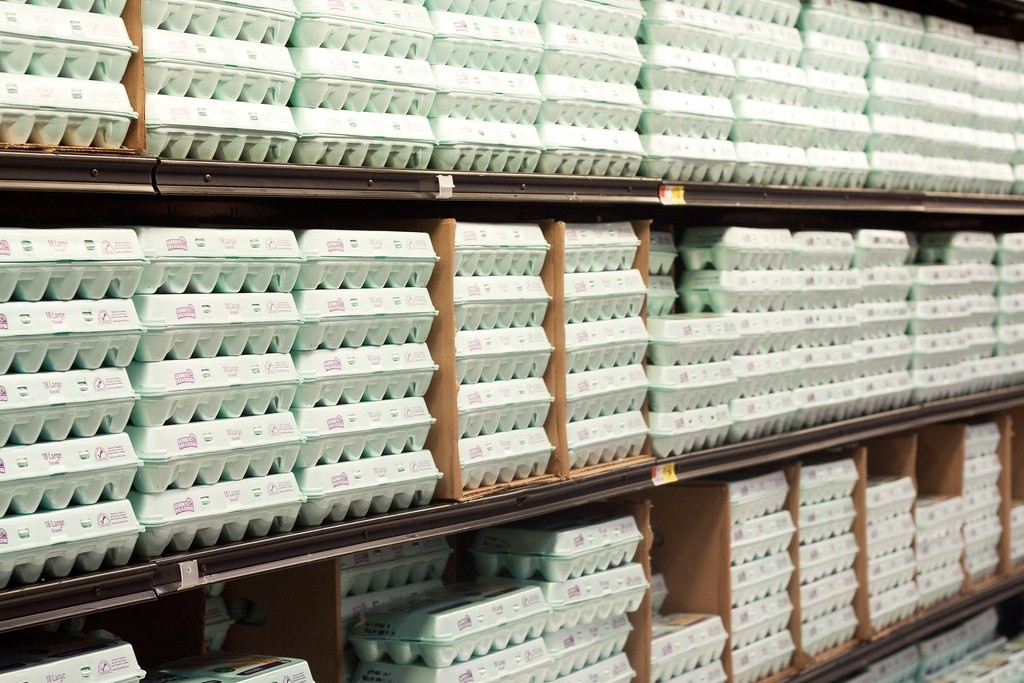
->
[0,0,1024,683]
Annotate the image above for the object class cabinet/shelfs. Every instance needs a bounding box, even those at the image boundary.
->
[919,189,1023,645]
[657,177,923,683]
[1,149,158,634]
[131,156,662,600]
